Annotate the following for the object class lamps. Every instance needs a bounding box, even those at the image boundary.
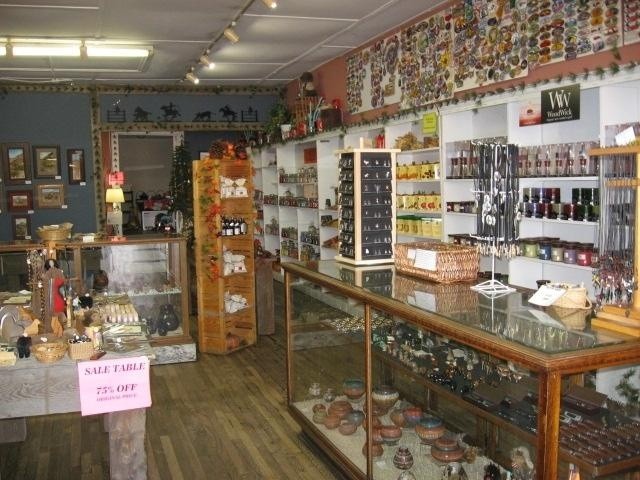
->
[105,188,126,211]
[183,1,278,85]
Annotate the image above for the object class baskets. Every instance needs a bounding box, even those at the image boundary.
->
[388,237,483,287]
[536,279,593,312]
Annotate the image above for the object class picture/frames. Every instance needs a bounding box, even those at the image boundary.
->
[6,189,34,214]
[2,142,33,185]
[32,144,62,179]
[66,148,86,185]
[37,182,65,210]
[12,214,32,240]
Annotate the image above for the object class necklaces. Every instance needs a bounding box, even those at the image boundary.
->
[467,141,521,261]
[590,143,636,309]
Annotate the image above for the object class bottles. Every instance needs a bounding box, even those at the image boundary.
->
[221,215,247,237]
[158,304,179,331]
[377,134,385,149]
[279,168,317,183]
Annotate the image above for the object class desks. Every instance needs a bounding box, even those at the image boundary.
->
[0,292,157,480]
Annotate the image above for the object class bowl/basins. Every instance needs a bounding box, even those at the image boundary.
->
[36,228,71,241]
[33,343,69,362]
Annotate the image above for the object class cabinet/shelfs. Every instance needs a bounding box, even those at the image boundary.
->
[0,229,199,365]
[192,158,257,356]
